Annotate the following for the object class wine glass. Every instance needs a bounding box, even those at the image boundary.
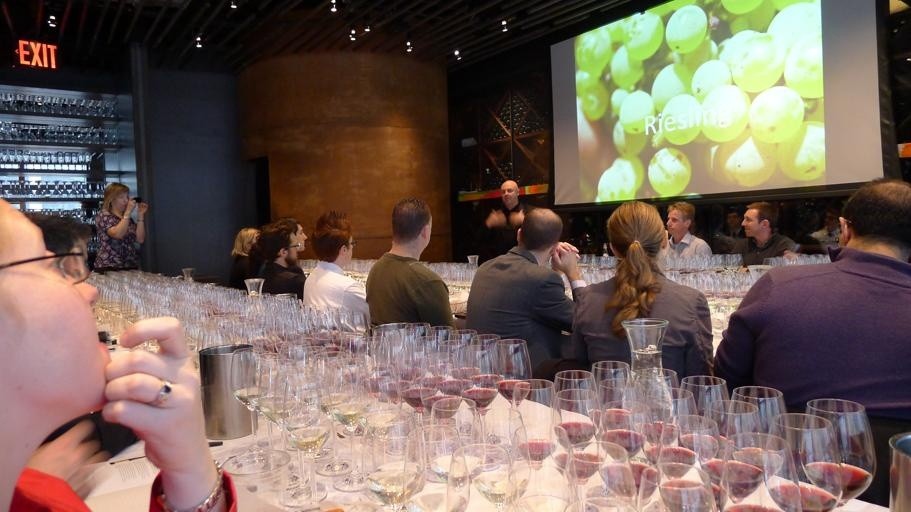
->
[544,252,617,293]
[220,309,878,512]
[0,91,123,119]
[664,249,832,331]
[0,181,109,201]
[87,270,373,379]
[17,207,101,226]
[344,257,378,284]
[420,255,479,292]
[87,234,100,253]
[0,149,93,169]
[299,259,320,275]
[0,121,120,146]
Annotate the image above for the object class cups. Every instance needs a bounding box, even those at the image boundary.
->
[888,431,911,512]
[193,343,260,440]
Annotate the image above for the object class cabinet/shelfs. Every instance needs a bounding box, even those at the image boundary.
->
[0,90,139,265]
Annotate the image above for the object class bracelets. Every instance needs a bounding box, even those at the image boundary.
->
[147,456,225,510]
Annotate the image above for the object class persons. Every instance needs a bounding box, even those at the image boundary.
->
[465,207,593,377]
[808,206,847,242]
[1,195,243,511]
[231,224,264,292]
[712,181,909,504]
[362,198,456,330]
[486,182,536,252]
[254,215,317,298]
[301,207,377,324]
[728,199,803,267]
[573,197,714,380]
[661,199,712,255]
[722,208,749,239]
[15,208,96,285]
[82,185,150,274]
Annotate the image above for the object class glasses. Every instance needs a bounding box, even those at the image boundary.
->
[285,242,303,250]
[346,239,359,248]
[0,242,92,286]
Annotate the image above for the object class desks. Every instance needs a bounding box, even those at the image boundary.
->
[72,322,898,511]
[696,295,744,359]
[304,266,470,314]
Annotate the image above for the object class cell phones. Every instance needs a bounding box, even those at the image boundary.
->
[133,197,142,204]
